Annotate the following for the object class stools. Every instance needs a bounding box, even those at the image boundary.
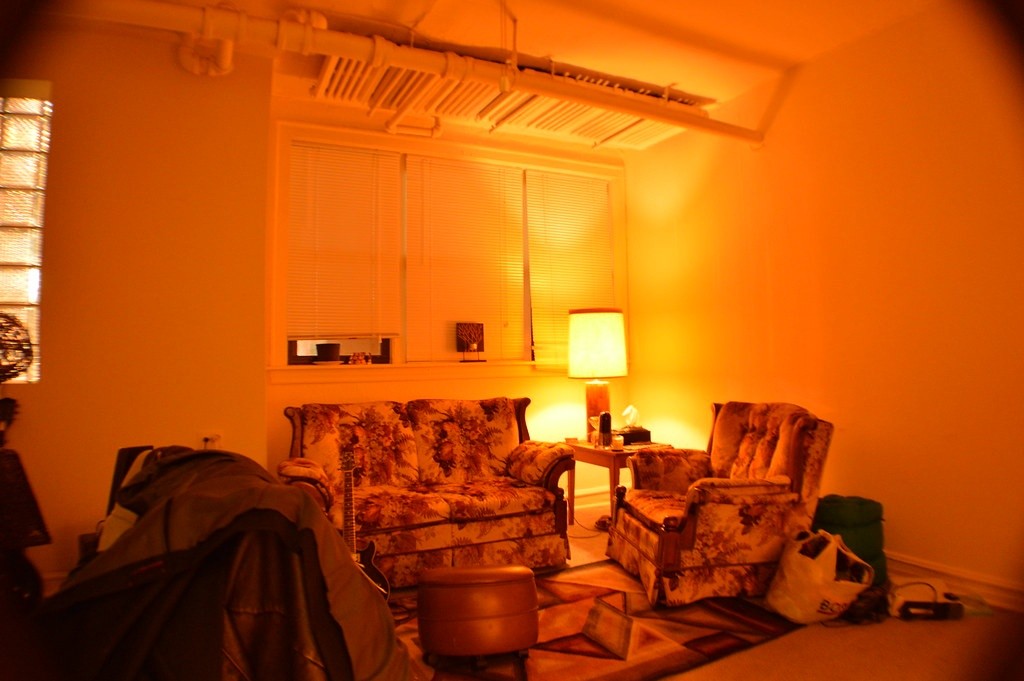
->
[418,566,538,681]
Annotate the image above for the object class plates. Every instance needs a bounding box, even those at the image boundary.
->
[312,361,344,365]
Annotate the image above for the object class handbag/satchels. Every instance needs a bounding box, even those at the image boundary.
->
[886,574,965,620]
[765,522,874,625]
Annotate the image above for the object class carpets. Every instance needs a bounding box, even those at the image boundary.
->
[388,559,807,681]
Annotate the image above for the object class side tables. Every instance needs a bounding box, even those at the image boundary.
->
[568,440,673,525]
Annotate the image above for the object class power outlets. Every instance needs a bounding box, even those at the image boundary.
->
[199,430,223,451]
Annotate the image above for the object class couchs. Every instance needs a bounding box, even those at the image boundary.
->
[606,401,835,609]
[277,398,575,590]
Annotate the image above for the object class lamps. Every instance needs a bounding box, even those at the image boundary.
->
[567,308,628,443]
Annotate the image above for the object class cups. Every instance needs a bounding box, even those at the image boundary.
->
[594,434,606,449]
[612,435,624,449]
[316,343,340,361]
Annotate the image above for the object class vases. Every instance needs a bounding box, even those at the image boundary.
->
[317,343,340,361]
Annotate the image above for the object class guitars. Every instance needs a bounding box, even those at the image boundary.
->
[339,449,392,606]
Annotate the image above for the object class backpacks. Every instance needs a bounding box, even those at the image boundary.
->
[812,494,889,623]
